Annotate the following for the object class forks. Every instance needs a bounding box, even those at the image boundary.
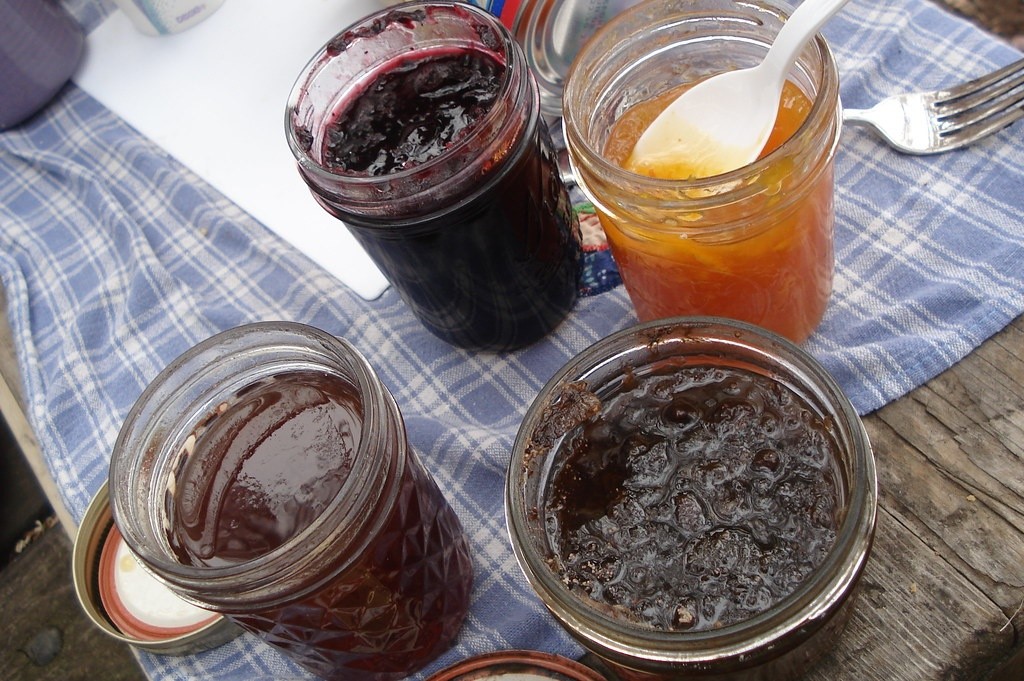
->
[843,57,1024,156]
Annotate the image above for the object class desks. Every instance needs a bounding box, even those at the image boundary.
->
[0,0,1024,680]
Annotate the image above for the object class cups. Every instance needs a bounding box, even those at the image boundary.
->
[1,0,88,133]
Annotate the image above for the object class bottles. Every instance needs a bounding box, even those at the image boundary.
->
[284,0,584,353]
[108,321,475,681]
[502,314,878,681]
[563,0,843,345]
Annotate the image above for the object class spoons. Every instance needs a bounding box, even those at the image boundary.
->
[626,0,852,221]
